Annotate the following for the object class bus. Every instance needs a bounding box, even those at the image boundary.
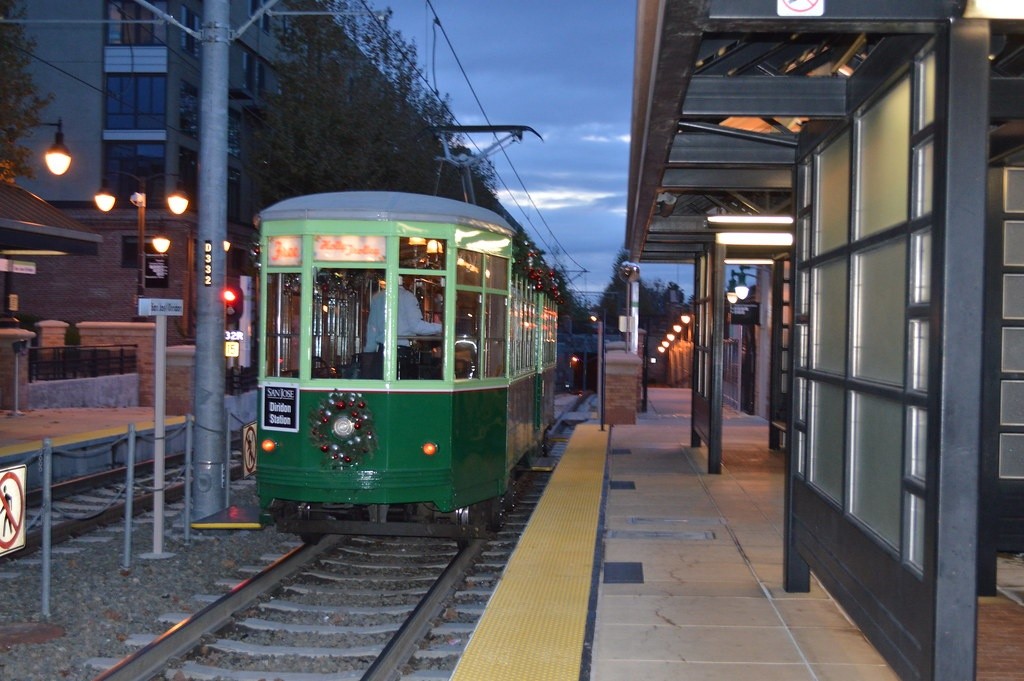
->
[252,125,560,540]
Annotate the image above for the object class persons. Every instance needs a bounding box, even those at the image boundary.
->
[360,260,442,380]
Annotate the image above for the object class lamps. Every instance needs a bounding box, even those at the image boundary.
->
[734,265,772,299]
[726,269,763,303]
[703,213,794,249]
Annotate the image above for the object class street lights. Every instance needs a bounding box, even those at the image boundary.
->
[92,169,189,323]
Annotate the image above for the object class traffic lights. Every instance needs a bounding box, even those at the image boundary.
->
[223,286,245,332]
